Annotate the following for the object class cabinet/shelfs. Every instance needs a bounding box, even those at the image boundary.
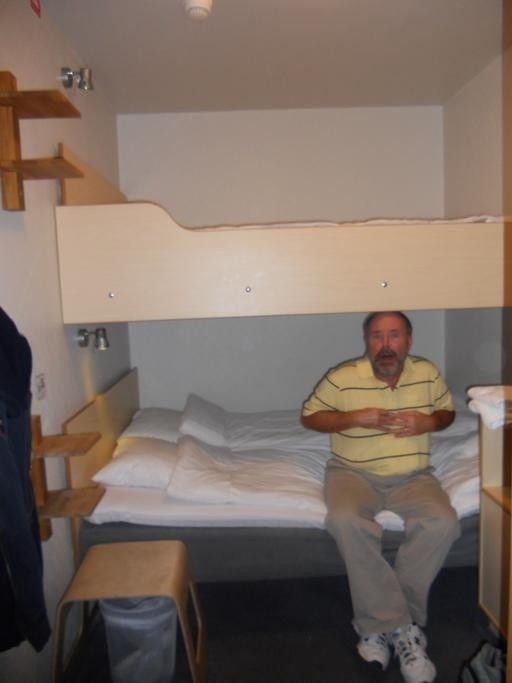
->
[28,414,108,540]
[0,69,82,210]
[477,414,511,640]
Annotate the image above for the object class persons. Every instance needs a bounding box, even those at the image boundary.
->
[300,315,462,683]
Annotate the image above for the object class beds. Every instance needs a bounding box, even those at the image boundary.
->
[59,368,480,587]
[55,139,511,323]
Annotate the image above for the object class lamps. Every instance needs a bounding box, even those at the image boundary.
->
[77,328,109,352]
[61,65,94,91]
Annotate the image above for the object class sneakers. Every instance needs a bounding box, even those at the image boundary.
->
[389,622,437,683]
[352,618,392,670]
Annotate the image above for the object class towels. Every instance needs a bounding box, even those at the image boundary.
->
[466,381,511,428]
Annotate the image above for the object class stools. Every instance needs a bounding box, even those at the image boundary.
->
[52,540,212,682]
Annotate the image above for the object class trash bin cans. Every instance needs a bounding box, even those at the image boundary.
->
[100,597,177,683]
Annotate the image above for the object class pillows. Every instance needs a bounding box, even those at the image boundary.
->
[92,406,181,489]
